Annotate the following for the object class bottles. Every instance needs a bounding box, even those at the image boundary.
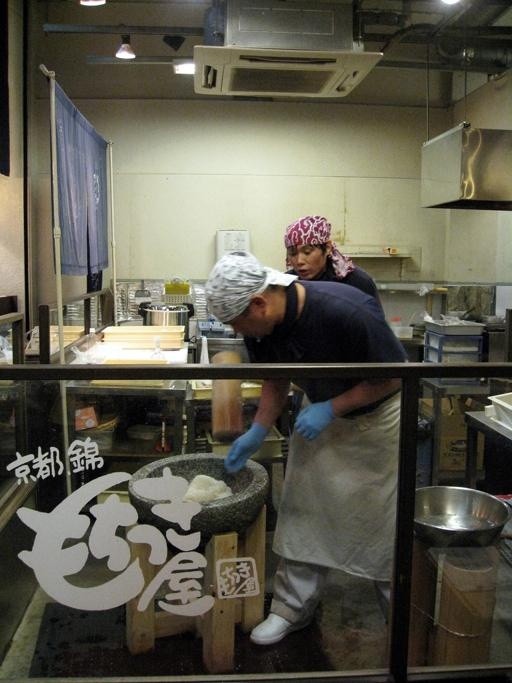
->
[390,317,402,327]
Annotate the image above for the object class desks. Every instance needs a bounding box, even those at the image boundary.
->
[464,411,512,488]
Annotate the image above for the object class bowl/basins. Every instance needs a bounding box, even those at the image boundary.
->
[449,309,505,323]
[413,484,511,548]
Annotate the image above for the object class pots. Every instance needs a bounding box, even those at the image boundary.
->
[143,307,190,341]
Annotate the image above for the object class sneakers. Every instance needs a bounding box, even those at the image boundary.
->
[249,613,312,645]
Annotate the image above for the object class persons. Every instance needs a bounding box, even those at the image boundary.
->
[204,249,410,646]
[283,215,381,303]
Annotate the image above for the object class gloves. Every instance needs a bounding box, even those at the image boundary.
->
[294,398,335,442]
[223,422,269,474]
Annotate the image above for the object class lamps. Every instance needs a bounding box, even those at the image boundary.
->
[115,34,136,59]
[172,58,194,76]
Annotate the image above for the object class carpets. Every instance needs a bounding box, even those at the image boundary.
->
[30,603,333,678]
